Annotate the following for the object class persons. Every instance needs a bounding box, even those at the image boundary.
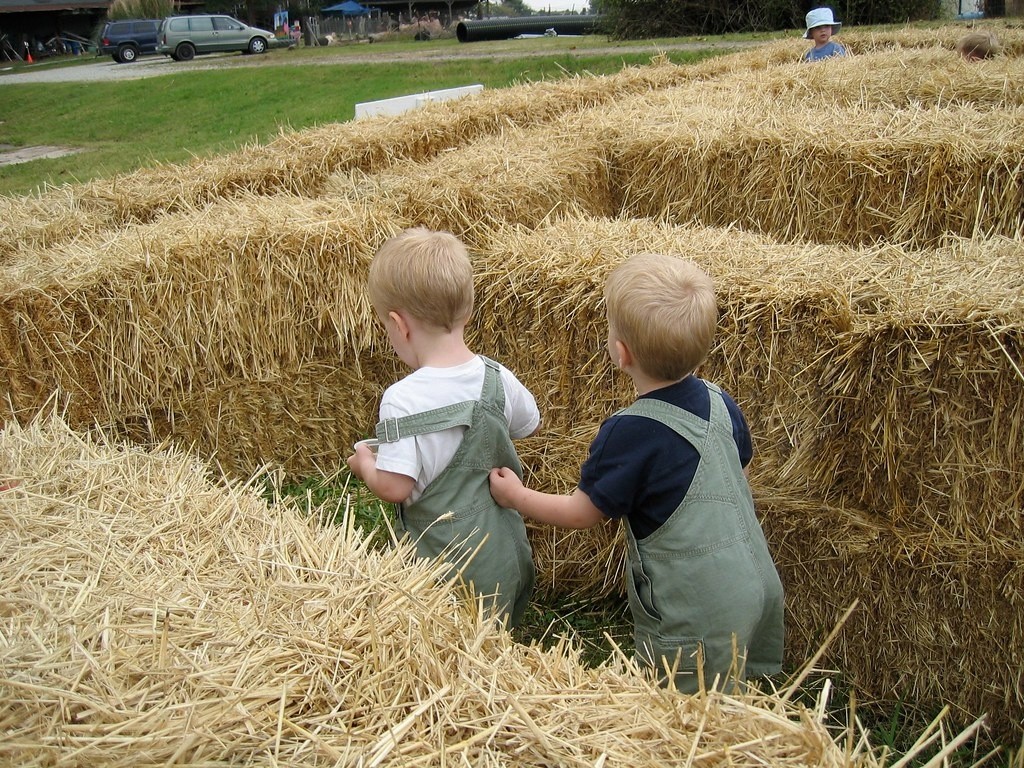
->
[956,31,998,59]
[490,253,785,702]
[346,17,353,40]
[803,8,846,61]
[347,228,540,638]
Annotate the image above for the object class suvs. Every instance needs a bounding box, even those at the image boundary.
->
[154,15,279,62]
[97,17,164,64]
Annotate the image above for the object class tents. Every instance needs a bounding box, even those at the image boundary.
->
[320,1,381,20]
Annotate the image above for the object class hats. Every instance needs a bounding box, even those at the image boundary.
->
[802,7,842,39]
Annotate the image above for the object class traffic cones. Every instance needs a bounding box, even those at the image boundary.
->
[27,54,33,64]
